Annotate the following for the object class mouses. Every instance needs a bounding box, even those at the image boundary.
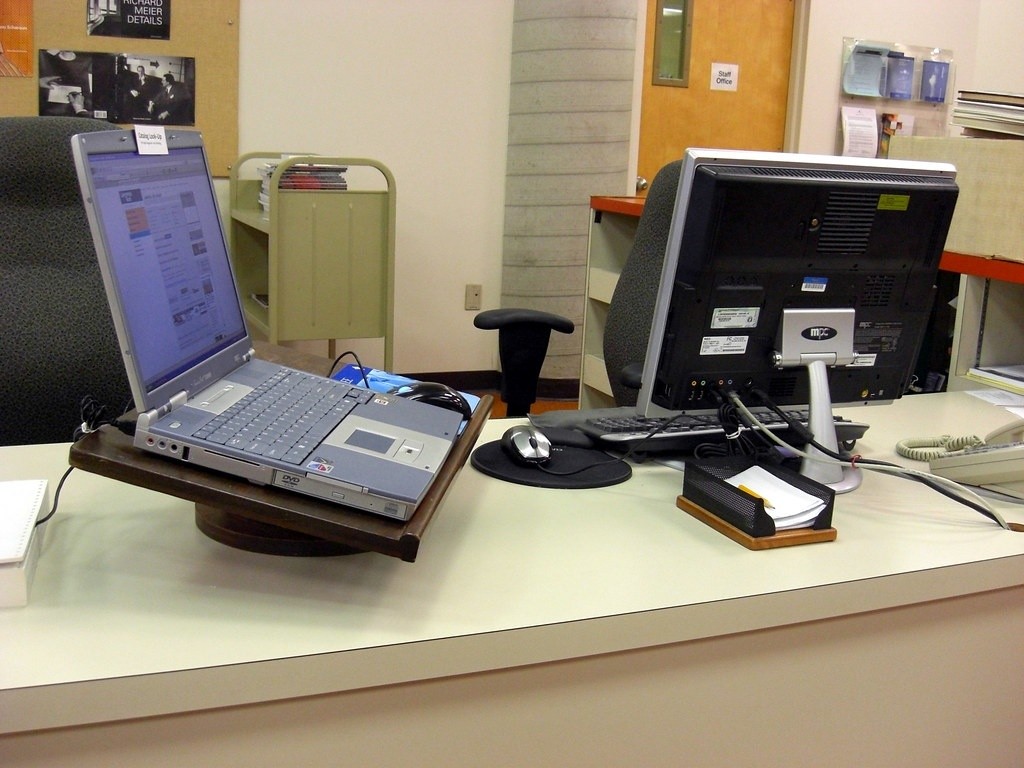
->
[385,382,472,422]
[502,425,554,468]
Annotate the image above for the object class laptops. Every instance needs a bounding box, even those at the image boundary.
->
[69,125,464,521]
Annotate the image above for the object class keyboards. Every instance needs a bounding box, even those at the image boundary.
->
[576,408,868,458]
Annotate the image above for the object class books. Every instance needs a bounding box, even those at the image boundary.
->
[252,294,269,310]
[256,162,347,221]
[971,366,1024,388]
[949,90,1024,136]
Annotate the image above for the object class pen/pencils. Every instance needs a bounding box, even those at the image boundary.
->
[723,479,776,510]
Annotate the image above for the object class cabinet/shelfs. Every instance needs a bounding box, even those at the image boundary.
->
[226,151,396,376]
[578,196,649,410]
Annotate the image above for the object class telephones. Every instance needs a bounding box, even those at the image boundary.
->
[930,418,1024,487]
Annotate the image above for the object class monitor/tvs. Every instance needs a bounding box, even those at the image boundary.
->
[634,149,962,495]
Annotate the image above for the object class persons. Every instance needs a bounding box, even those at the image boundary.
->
[124,66,182,122]
[39,77,92,117]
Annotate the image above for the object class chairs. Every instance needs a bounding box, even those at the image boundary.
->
[0,116,138,447]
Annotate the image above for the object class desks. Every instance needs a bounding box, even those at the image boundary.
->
[0,389,1024,768]
